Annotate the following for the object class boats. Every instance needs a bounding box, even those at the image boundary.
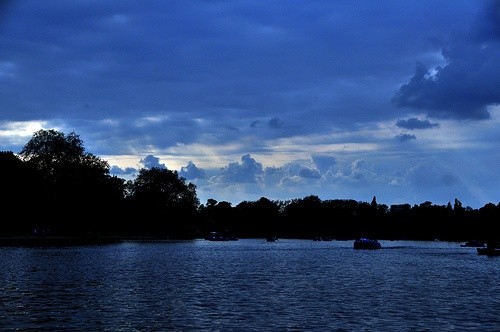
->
[477,243,500,255]
[353,238,381,249]
[206,232,333,242]
